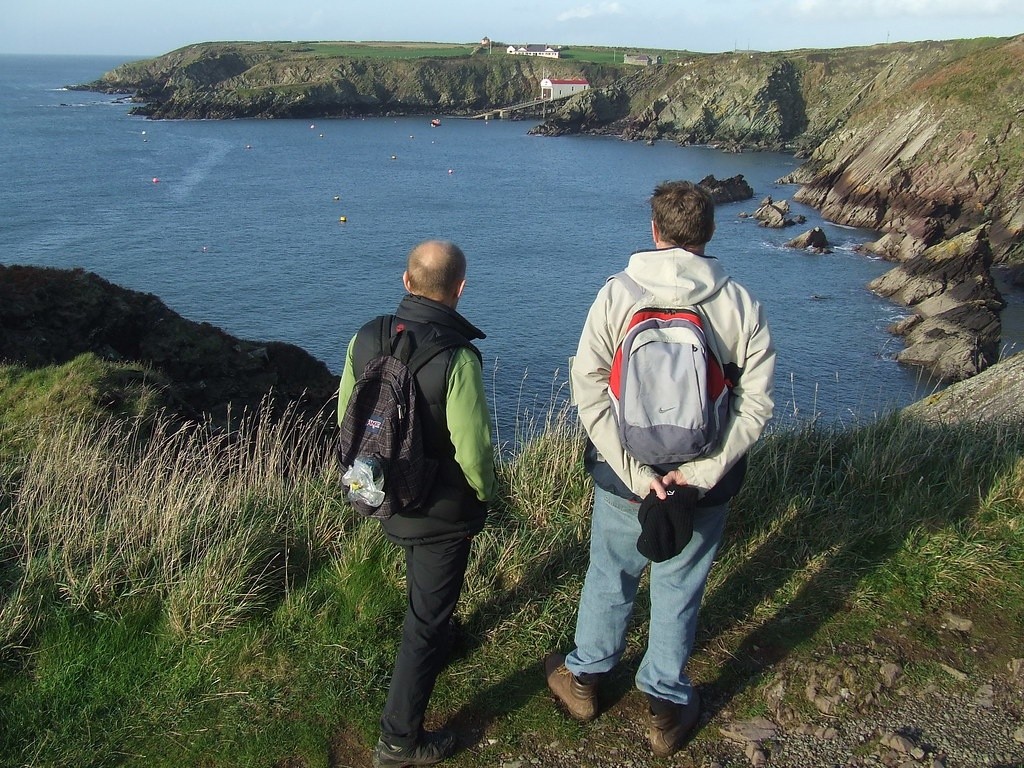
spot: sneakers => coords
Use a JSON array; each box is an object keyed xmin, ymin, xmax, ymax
[
  {"xmin": 543, "ymin": 654, "xmax": 598, "ymax": 721},
  {"xmin": 372, "ymin": 731, "xmax": 457, "ymax": 768},
  {"xmin": 648, "ymin": 687, "xmax": 700, "ymax": 759}
]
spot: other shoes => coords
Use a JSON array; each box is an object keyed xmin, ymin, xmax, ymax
[{"xmin": 448, "ymin": 619, "xmax": 455, "ymax": 647}]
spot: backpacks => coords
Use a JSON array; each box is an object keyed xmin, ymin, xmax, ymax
[
  {"xmin": 605, "ymin": 270, "xmax": 743, "ymax": 466},
  {"xmin": 336, "ymin": 315, "xmax": 483, "ymax": 520}
]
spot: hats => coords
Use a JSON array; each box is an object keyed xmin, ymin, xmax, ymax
[{"xmin": 636, "ymin": 485, "xmax": 699, "ymax": 563}]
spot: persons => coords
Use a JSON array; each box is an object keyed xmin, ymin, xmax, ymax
[
  {"xmin": 338, "ymin": 241, "xmax": 499, "ymax": 768},
  {"xmin": 545, "ymin": 181, "xmax": 775, "ymax": 757}
]
